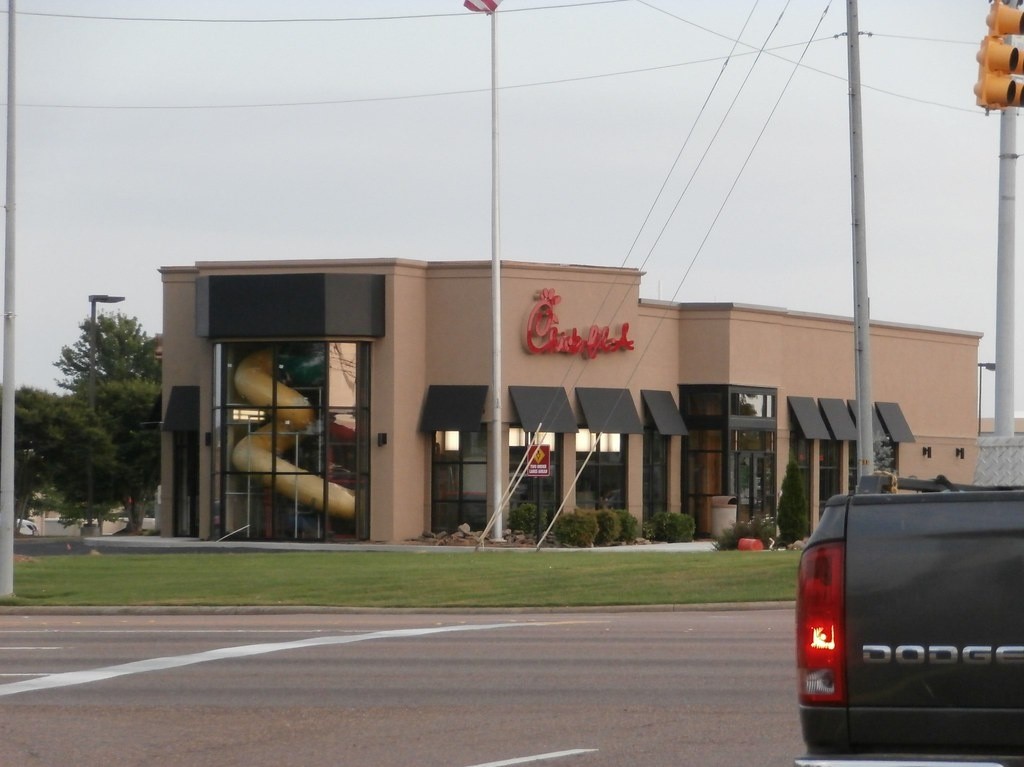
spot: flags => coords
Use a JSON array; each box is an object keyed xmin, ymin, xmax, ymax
[{"xmin": 464, "ymin": 0, "xmax": 504, "ymax": 15}]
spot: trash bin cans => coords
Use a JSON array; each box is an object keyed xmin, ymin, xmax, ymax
[{"xmin": 710, "ymin": 496, "xmax": 738, "ymax": 540}]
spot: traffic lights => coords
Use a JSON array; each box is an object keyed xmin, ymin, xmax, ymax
[{"xmin": 974, "ymin": 0, "xmax": 1024, "ymax": 114}]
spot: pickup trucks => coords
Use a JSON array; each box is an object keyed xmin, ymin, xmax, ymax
[{"xmin": 790, "ymin": 472, "xmax": 1024, "ymax": 765}]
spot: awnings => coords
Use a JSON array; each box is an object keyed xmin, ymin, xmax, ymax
[
  {"xmin": 641, "ymin": 390, "xmax": 689, "ymax": 435},
  {"xmin": 575, "ymin": 387, "xmax": 645, "ymax": 434},
  {"xmin": 875, "ymin": 402, "xmax": 916, "ymax": 443},
  {"xmin": 421, "ymin": 384, "xmax": 489, "ymax": 431},
  {"xmin": 818, "ymin": 398, "xmax": 857, "ymax": 440},
  {"xmin": 787, "ymin": 396, "xmax": 831, "ymax": 440},
  {"xmin": 162, "ymin": 386, "xmax": 200, "ymax": 431},
  {"xmin": 847, "ymin": 400, "xmax": 888, "ymax": 441},
  {"xmin": 508, "ymin": 385, "xmax": 579, "ymax": 432}
]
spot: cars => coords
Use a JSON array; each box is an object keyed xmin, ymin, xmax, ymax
[{"xmin": 15, "ymin": 519, "xmax": 38, "ymax": 536}]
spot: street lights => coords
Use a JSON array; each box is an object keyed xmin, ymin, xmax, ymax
[{"xmin": 84, "ymin": 295, "xmax": 126, "ymax": 527}]
